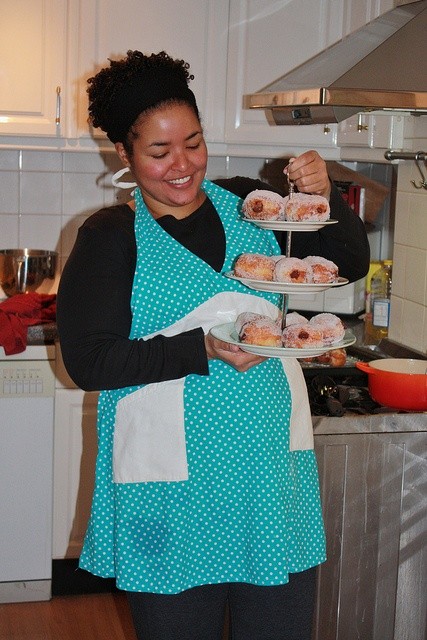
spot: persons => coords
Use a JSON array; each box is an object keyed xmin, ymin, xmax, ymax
[{"xmin": 57, "ymin": 51, "xmax": 370, "ymax": 638}]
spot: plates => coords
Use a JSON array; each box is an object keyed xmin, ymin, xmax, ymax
[
  {"xmin": 220, "ymin": 271, "xmax": 349, "ymax": 298},
  {"xmin": 241, "ymin": 216, "xmax": 340, "ymax": 236},
  {"xmin": 208, "ymin": 321, "xmax": 355, "ymax": 359}
]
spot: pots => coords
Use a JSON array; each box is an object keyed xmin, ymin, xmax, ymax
[
  {"xmin": 0, "ymin": 249, "xmax": 60, "ymax": 297},
  {"xmin": 352, "ymin": 358, "xmax": 426, "ymax": 411}
]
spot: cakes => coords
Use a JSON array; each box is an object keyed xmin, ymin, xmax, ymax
[
  {"xmin": 242, "ymin": 189, "xmax": 330, "ymax": 222},
  {"xmin": 235, "ymin": 311, "xmax": 346, "ymax": 349},
  {"xmin": 235, "ymin": 253, "xmax": 339, "ymax": 284}
]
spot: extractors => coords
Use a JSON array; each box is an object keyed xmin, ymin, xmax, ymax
[{"xmin": 244, "ymin": 1, "xmax": 427, "ymax": 128}]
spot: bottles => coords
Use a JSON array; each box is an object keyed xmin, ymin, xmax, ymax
[{"xmin": 365, "ymin": 254, "xmax": 392, "ymax": 333}]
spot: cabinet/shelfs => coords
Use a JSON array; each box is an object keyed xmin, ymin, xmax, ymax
[
  {"xmin": 53, "ymin": 392, "xmax": 101, "ymax": 560},
  {"xmin": 229, "ymin": 1, "xmax": 405, "ymax": 164},
  {"xmin": 385, "ymin": 149, "xmax": 426, "ymax": 189},
  {"xmin": 1, "ymin": 0, "xmax": 230, "ymax": 158}
]
